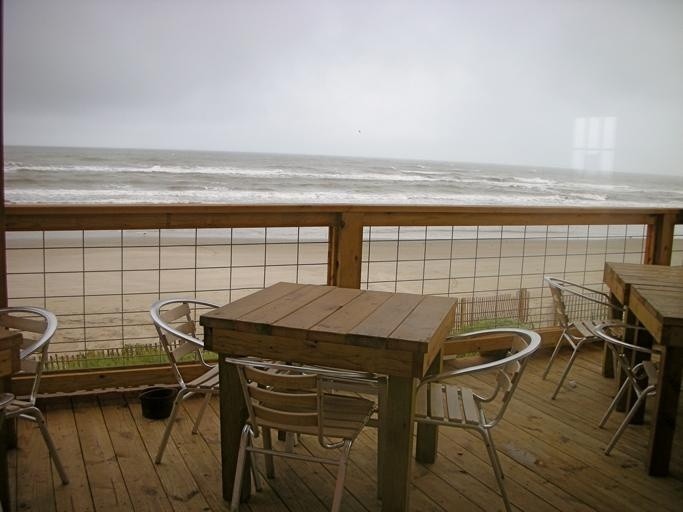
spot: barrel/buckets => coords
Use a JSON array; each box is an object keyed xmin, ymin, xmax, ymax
[{"xmin": 138, "ymin": 385, "xmax": 178, "ymax": 420}]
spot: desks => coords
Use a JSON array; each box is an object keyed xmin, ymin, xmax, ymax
[
  {"xmin": 198, "ymin": 281, "xmax": 458, "ymax": 512},
  {"xmin": 601, "ymin": 261, "xmax": 682, "ymax": 477}
]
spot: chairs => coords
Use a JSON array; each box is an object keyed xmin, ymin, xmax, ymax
[
  {"xmin": 542, "ymin": 277, "xmax": 682, "ymax": 456},
  {"xmin": 0, "ymin": 305, "xmax": 69, "ymax": 486}
]
